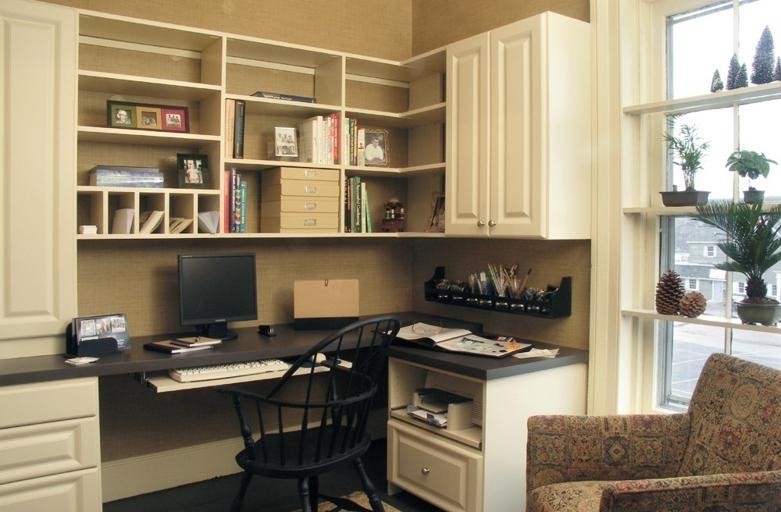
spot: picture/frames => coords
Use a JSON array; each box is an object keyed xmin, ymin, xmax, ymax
[{"xmin": 1, "ymin": 311, "xmax": 591, "ymax": 512}]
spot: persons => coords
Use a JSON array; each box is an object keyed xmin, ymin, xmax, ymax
[
  {"xmin": 142, "ymin": 112, "xmax": 156, "ymax": 126},
  {"xmin": 364, "ymin": 137, "xmax": 385, "ymax": 161},
  {"xmin": 185, "ymin": 163, "xmax": 202, "ymax": 183},
  {"xmin": 277, "ymin": 132, "xmax": 298, "ymax": 157},
  {"xmin": 167, "ymin": 115, "xmax": 181, "ymax": 127},
  {"xmin": 116, "ymin": 110, "xmax": 131, "ymax": 124},
  {"xmin": 113, "ymin": 319, "xmax": 125, "ymax": 329},
  {"xmin": 98, "ymin": 319, "xmax": 108, "ymax": 334}
]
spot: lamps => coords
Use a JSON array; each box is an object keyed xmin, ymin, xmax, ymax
[
  {"xmin": 212, "ymin": 315, "xmax": 402, "ymax": 511},
  {"xmin": 525, "ymin": 353, "xmax": 781, "ymax": 511}
]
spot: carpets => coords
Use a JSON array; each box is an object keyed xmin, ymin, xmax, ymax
[{"xmin": 298, "ymin": 488, "xmax": 403, "ymax": 512}]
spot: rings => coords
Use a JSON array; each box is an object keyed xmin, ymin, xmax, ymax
[{"xmin": 486, "ymin": 263, "xmax": 532, "ymax": 298}]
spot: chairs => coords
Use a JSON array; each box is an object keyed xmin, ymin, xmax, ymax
[
  {"xmin": 525, "ymin": 353, "xmax": 781, "ymax": 511},
  {"xmin": 212, "ymin": 315, "xmax": 402, "ymax": 511}
]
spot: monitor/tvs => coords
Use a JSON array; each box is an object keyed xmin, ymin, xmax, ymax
[
  {"xmin": 198, "ymin": 211, "xmax": 220, "ymax": 233},
  {"xmin": 344, "ymin": 175, "xmax": 372, "ymax": 234},
  {"xmin": 233, "ymin": 100, "xmax": 246, "ymax": 160},
  {"xmin": 298, "ymin": 112, "xmax": 365, "ymax": 165},
  {"xmin": 142, "ymin": 339, "xmax": 213, "ymax": 355},
  {"xmin": 224, "ymin": 98, "xmax": 235, "ymax": 159},
  {"xmin": 251, "ymin": 91, "xmax": 316, "ymax": 103},
  {"xmin": 170, "ymin": 335, "xmax": 224, "ymax": 348},
  {"xmin": 408, "ymin": 408, "xmax": 447, "ymax": 427},
  {"xmin": 139, "ymin": 210, "xmax": 165, "ymax": 234},
  {"xmin": 382, "ymin": 321, "xmax": 533, "ymax": 360},
  {"xmin": 89, "ymin": 165, "xmax": 165, "ymax": 187},
  {"xmin": 111, "ymin": 208, "xmax": 134, "ymax": 235},
  {"xmin": 225, "ymin": 167, "xmax": 248, "ymax": 233}
]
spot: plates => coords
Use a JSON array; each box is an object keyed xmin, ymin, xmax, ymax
[{"xmin": 618, "ymin": 0, "xmax": 781, "ymax": 335}]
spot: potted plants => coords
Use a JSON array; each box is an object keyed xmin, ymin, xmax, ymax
[
  {"xmin": 689, "ymin": 200, "xmax": 781, "ymax": 327},
  {"xmin": 661, "ymin": 110, "xmax": 713, "ymax": 208},
  {"xmin": 726, "ymin": 148, "xmax": 780, "ymax": 205}
]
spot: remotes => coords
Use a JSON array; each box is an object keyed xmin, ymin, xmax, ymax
[{"xmin": 310, "ymin": 352, "xmax": 325, "ymax": 365}]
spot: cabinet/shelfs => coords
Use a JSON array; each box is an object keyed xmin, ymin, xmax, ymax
[
  {"xmin": 3, "ymin": 2, "xmax": 78, "ymax": 360},
  {"xmin": 316, "ymin": 53, "xmax": 449, "ymax": 235},
  {"xmin": 618, "ymin": 0, "xmax": 781, "ymax": 335},
  {"xmin": 77, "ymin": 12, "xmax": 222, "ymax": 237},
  {"xmin": 446, "ymin": 12, "xmax": 593, "ymax": 240},
  {"xmin": 201, "ymin": 34, "xmax": 344, "ymax": 237}
]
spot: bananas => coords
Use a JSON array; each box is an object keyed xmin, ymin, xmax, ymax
[{"xmin": 168, "ymin": 359, "xmax": 290, "ymax": 383}]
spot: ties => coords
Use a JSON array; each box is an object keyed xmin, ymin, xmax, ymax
[{"xmin": 177, "ymin": 253, "xmax": 258, "ymax": 341}]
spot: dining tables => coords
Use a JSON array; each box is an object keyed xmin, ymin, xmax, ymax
[
  {"xmin": 726, "ymin": 148, "xmax": 780, "ymax": 205},
  {"xmin": 661, "ymin": 110, "xmax": 713, "ymax": 208},
  {"xmin": 689, "ymin": 200, "xmax": 781, "ymax": 327}
]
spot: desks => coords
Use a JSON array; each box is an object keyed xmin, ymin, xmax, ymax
[{"xmin": 1, "ymin": 311, "xmax": 591, "ymax": 512}]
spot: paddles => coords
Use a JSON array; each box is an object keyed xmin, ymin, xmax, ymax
[{"xmin": 298, "ymin": 488, "xmax": 403, "ymax": 512}]
